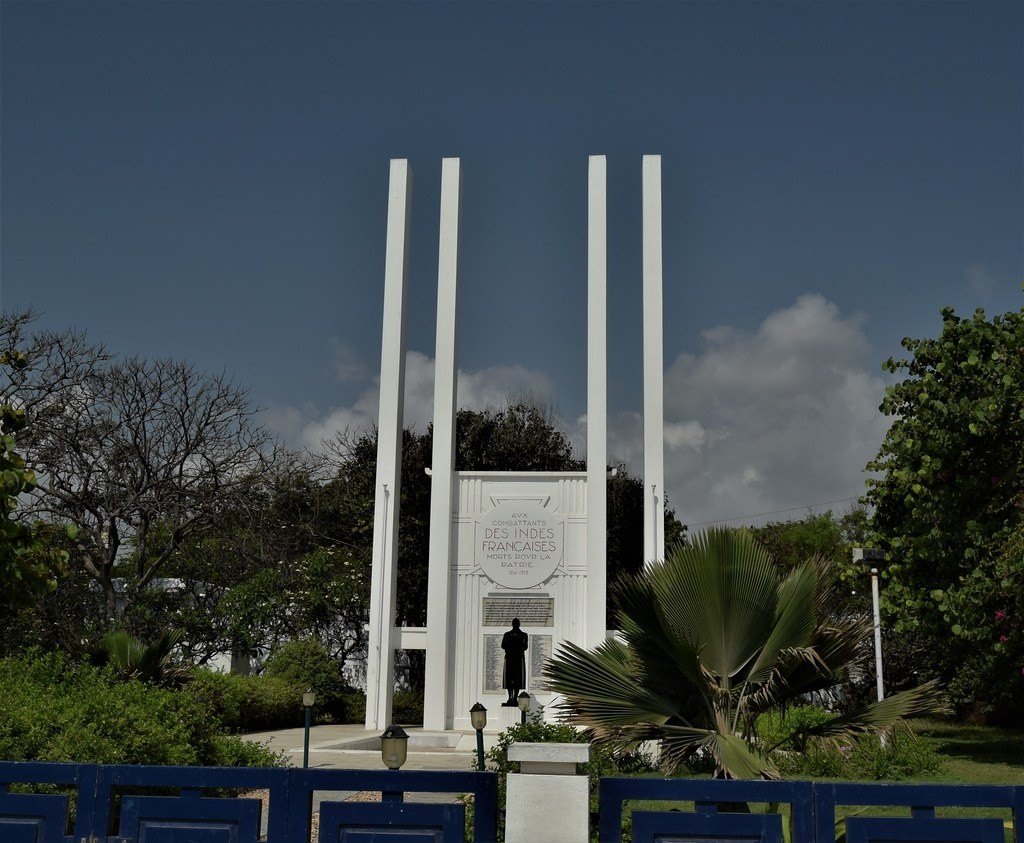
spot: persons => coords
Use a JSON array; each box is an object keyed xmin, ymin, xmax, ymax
[{"xmin": 501, "ymin": 617, "xmax": 528, "ymax": 707}]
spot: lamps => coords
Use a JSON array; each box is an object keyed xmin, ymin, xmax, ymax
[
  {"xmin": 469, "ymin": 702, "xmax": 488, "ymax": 731},
  {"xmin": 517, "ymin": 691, "xmax": 531, "ymax": 711},
  {"xmin": 302, "ymin": 687, "xmax": 316, "ymax": 706},
  {"xmin": 380, "ymin": 722, "xmax": 410, "ymax": 768}
]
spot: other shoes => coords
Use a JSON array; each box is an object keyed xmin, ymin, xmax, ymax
[{"xmin": 507, "ymin": 698, "xmax": 518, "ymax": 705}]
